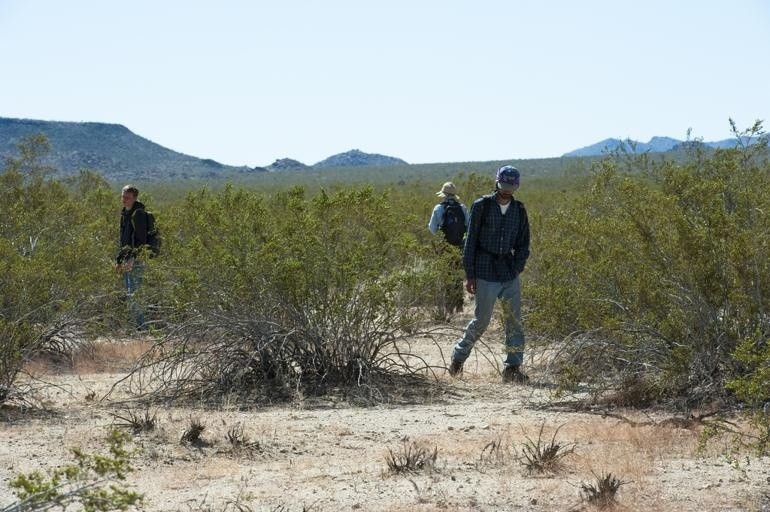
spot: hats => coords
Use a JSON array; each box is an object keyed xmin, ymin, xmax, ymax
[
  {"xmin": 436, "ymin": 181, "xmax": 460, "ymax": 200},
  {"xmin": 495, "ymin": 165, "xmax": 520, "ymax": 189}
]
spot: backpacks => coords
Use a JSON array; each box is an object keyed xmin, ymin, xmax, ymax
[
  {"xmin": 439, "ymin": 201, "xmax": 467, "ymax": 246},
  {"xmin": 131, "ymin": 208, "xmax": 162, "ymax": 259}
]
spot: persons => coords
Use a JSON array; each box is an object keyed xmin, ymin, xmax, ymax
[
  {"xmin": 444, "ymin": 162, "xmax": 533, "ymax": 387},
  {"xmin": 111, "ymin": 183, "xmax": 151, "ymax": 333},
  {"xmin": 426, "ymin": 179, "xmax": 471, "ymax": 318}
]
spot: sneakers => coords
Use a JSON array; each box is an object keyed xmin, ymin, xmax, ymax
[
  {"xmin": 501, "ymin": 368, "xmax": 531, "ymax": 383},
  {"xmin": 448, "ymin": 354, "xmax": 464, "ymax": 380}
]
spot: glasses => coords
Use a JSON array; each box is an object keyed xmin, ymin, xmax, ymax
[{"xmin": 497, "ymin": 183, "xmax": 517, "ymax": 191}]
